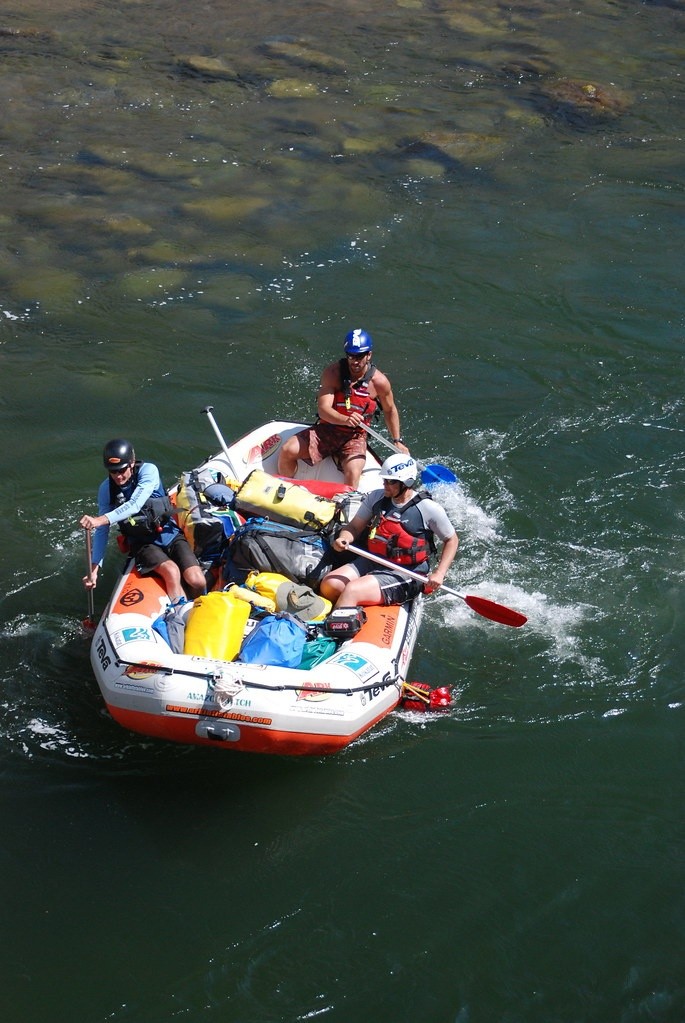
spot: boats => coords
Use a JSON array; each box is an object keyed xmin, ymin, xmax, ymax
[{"xmin": 87, "ymin": 419, "xmax": 427, "ymax": 756}]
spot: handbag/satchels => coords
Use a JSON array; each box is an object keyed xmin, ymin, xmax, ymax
[{"xmin": 155, "ymin": 466, "xmax": 368, "ymax": 670}]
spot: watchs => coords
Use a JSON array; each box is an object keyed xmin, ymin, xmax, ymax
[{"xmin": 393, "ymin": 438, "xmax": 403, "ymax": 443}]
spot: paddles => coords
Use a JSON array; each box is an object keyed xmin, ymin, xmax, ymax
[
  {"xmin": 201, "ymin": 405, "xmax": 246, "ymax": 484},
  {"xmin": 357, "ymin": 421, "xmax": 458, "ymax": 485},
  {"xmin": 81, "ymin": 528, "xmax": 100, "ymax": 629},
  {"xmin": 344, "ymin": 542, "xmax": 528, "ymax": 628}
]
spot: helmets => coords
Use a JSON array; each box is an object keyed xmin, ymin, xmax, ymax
[
  {"xmin": 380, "ymin": 453, "xmax": 416, "ymax": 488},
  {"xmin": 103, "ymin": 439, "xmax": 134, "ymax": 470},
  {"xmin": 344, "ymin": 329, "xmax": 372, "ymax": 354}
]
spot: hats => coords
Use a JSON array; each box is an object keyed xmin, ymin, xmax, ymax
[{"xmin": 277, "ymin": 582, "xmax": 326, "ymax": 621}]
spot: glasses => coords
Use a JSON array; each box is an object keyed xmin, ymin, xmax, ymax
[
  {"xmin": 384, "ymin": 479, "xmax": 398, "ymax": 484},
  {"xmin": 109, "ymin": 465, "xmax": 129, "ymax": 474},
  {"xmin": 346, "ymin": 354, "xmax": 366, "ymax": 360}
]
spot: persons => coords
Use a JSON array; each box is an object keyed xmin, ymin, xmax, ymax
[
  {"xmin": 319, "ymin": 452, "xmax": 460, "ymax": 612},
  {"xmin": 79, "ymin": 438, "xmax": 208, "ymax": 606},
  {"xmin": 277, "ymin": 328, "xmax": 411, "ymax": 491}
]
